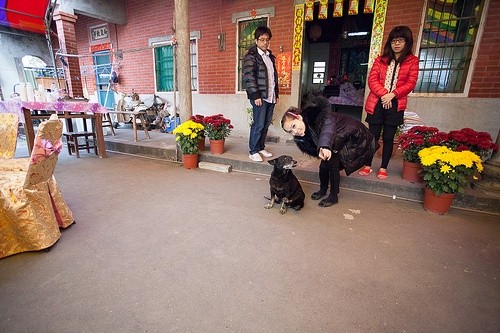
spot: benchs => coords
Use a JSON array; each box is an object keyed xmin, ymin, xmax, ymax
[{"xmin": 101, "ymin": 110, "xmax": 150, "ymax": 143}]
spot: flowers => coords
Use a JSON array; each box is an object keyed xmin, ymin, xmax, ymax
[
  {"xmin": 416, "ymin": 146, "xmax": 485, "ymax": 197},
  {"xmin": 189, "ymin": 114, "xmax": 205, "ymax": 137},
  {"xmin": 398, "ymin": 127, "xmax": 448, "ymax": 163},
  {"xmin": 203, "ymin": 114, "xmax": 234, "ymax": 140},
  {"xmin": 446, "ymin": 127, "xmax": 496, "ymax": 180},
  {"xmin": 172, "ymin": 120, "xmax": 205, "ymax": 154}
]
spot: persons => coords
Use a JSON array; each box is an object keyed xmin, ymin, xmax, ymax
[
  {"xmin": 281, "ymin": 96, "xmax": 376, "ymax": 207},
  {"xmin": 359, "ymin": 26, "xmax": 419, "ymax": 179},
  {"xmin": 242, "ymin": 27, "xmax": 279, "ymax": 162}
]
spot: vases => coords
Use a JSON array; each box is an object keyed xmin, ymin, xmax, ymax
[
  {"xmin": 183, "ymin": 153, "xmax": 199, "ymax": 168},
  {"xmin": 209, "ymin": 138, "xmax": 225, "ymax": 154},
  {"xmin": 198, "ymin": 137, "xmax": 206, "ymax": 151},
  {"xmin": 402, "ymin": 161, "xmax": 426, "ymax": 184},
  {"xmin": 423, "ymin": 186, "xmax": 456, "ymax": 215}
]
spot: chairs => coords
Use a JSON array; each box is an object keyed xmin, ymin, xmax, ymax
[
  {"xmin": 0, "ymin": 112, "xmax": 98, "ymax": 258},
  {"xmin": 328, "ymin": 81, "xmax": 366, "ymax": 119}
]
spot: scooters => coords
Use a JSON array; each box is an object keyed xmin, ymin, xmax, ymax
[{"xmin": 116, "ymin": 89, "xmax": 168, "ymax": 131}]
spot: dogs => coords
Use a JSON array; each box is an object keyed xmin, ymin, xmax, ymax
[{"xmin": 264, "ymin": 155, "xmax": 305, "ymax": 214}]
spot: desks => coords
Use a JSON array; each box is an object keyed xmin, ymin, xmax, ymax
[{"xmin": 0, "ymin": 100, "xmax": 107, "ymax": 160}]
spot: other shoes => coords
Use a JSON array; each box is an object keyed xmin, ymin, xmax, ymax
[
  {"xmin": 259, "ymin": 150, "xmax": 273, "ymax": 157},
  {"xmin": 318, "ymin": 198, "xmax": 338, "ymax": 207},
  {"xmin": 311, "ymin": 191, "xmax": 326, "ymax": 200},
  {"xmin": 249, "ymin": 153, "xmax": 263, "ymax": 161}
]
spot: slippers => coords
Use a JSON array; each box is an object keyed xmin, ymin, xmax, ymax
[
  {"xmin": 377, "ymin": 171, "xmax": 388, "ymax": 179},
  {"xmin": 358, "ymin": 168, "xmax": 373, "ymax": 176}
]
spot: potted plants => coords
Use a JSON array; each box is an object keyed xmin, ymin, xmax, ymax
[{"xmin": 378, "ymin": 125, "xmax": 405, "ymax": 158}]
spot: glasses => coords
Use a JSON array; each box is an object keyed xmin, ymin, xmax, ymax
[
  {"xmin": 257, "ymin": 38, "xmax": 270, "ymax": 42},
  {"xmin": 390, "ymin": 38, "xmax": 405, "ymax": 44}
]
